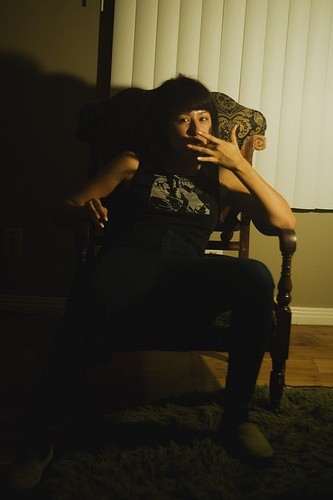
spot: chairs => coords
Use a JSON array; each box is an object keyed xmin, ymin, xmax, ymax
[{"xmin": 51, "ymin": 84, "xmax": 299, "ymax": 415}]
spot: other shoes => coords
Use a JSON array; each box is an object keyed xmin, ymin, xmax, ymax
[
  {"xmin": 219, "ymin": 414, "xmax": 273, "ymax": 460},
  {"xmin": 6, "ymin": 443, "xmax": 53, "ymax": 490}
]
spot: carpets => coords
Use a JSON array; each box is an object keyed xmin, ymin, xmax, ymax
[{"xmin": 0, "ymin": 383, "xmax": 333, "ymax": 500}]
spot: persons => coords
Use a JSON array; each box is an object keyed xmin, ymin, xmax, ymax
[{"xmin": 5, "ymin": 74, "xmax": 294, "ymax": 491}]
